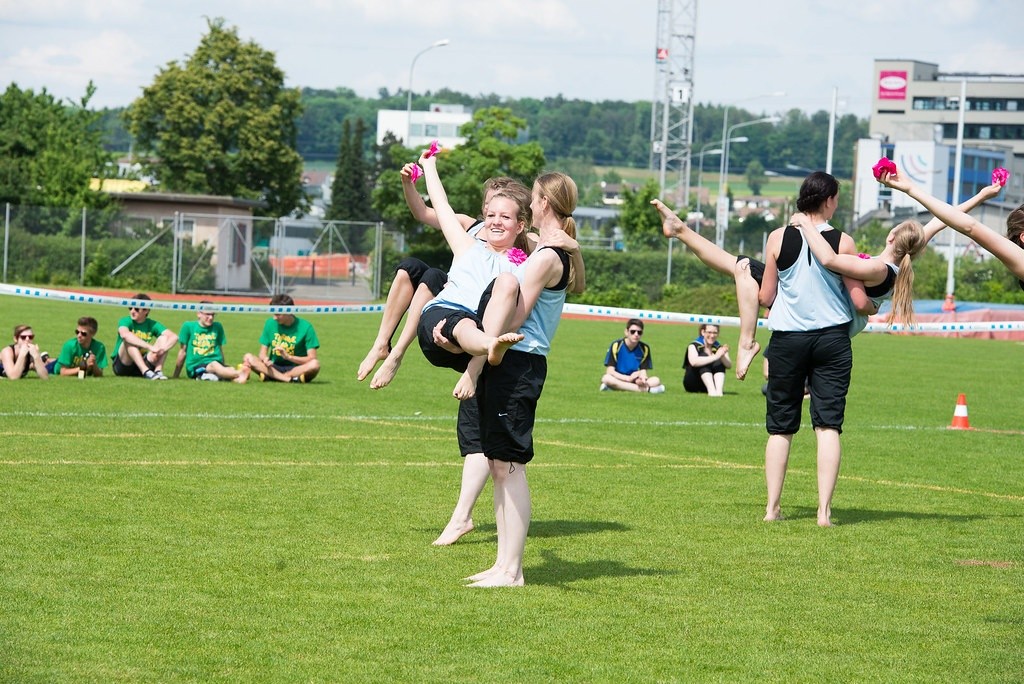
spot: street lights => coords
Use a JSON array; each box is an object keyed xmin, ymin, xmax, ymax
[
  {"xmin": 716, "ymin": 115, "xmax": 782, "ymax": 252},
  {"xmin": 404, "ymin": 39, "xmax": 452, "ymax": 146},
  {"xmin": 694, "ymin": 135, "xmax": 749, "ymax": 234}
]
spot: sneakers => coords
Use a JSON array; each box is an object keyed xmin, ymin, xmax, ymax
[
  {"xmin": 145, "ymin": 370, "xmax": 158, "ymax": 379},
  {"xmin": 158, "ymin": 372, "xmax": 167, "ymax": 379}
]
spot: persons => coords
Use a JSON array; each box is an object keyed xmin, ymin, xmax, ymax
[
  {"xmin": 682, "ymin": 324, "xmax": 732, "ymax": 397},
  {"xmin": 243, "ymin": 294, "xmax": 320, "ymax": 382},
  {"xmin": 650, "ymin": 166, "xmax": 1010, "ymax": 381},
  {"xmin": 758, "ymin": 171, "xmax": 878, "ymax": 527},
  {"xmin": 0, "ymin": 325, "xmax": 50, "ymax": 380},
  {"xmin": 169, "ymin": 300, "xmax": 252, "ymax": 384},
  {"xmin": 111, "ymin": 294, "xmax": 179, "ymax": 380},
  {"xmin": 356, "ymin": 142, "xmax": 587, "ymax": 588},
  {"xmin": 599, "ymin": 318, "xmax": 666, "ymax": 394},
  {"xmin": 30, "ymin": 317, "xmax": 110, "ymax": 379},
  {"xmin": 872, "ymin": 157, "xmax": 1024, "ymax": 294}
]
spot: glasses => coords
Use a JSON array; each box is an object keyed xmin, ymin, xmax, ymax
[
  {"xmin": 129, "ymin": 307, "xmax": 148, "ymax": 312},
  {"xmin": 75, "ymin": 329, "xmax": 88, "ymax": 337},
  {"xmin": 629, "ymin": 330, "xmax": 643, "ymax": 336},
  {"xmin": 19, "ymin": 335, "xmax": 34, "ymax": 340}
]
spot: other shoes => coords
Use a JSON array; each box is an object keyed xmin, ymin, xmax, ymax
[
  {"xmin": 294, "ymin": 374, "xmax": 307, "ymax": 384},
  {"xmin": 41, "ymin": 352, "xmax": 49, "ymax": 361},
  {"xmin": 259, "ymin": 372, "xmax": 270, "ymax": 381}
]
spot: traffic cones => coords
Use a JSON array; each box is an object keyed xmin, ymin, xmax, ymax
[{"xmin": 946, "ymin": 393, "xmax": 976, "ymax": 430}]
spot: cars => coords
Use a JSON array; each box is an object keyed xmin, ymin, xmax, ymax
[{"xmin": 320, "ymin": 172, "xmax": 334, "ymax": 207}]
